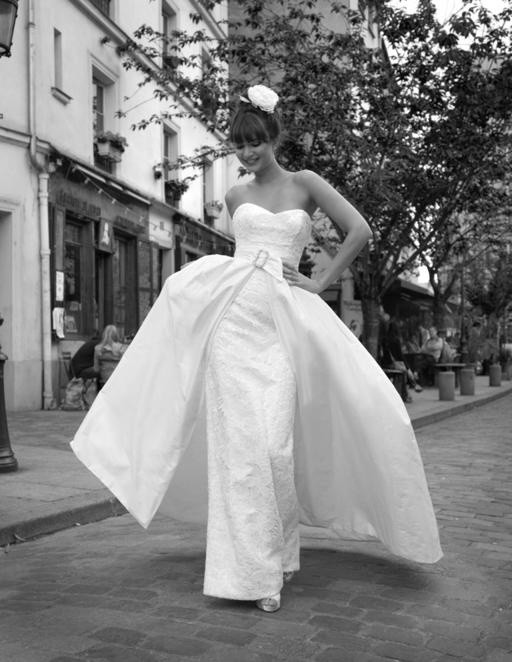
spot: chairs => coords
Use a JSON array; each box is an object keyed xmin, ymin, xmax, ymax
[{"xmin": 61, "ymin": 351, "xmax": 120, "ymax": 409}]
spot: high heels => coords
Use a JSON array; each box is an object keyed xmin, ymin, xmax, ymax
[{"xmin": 255, "ymin": 572, "xmax": 295, "ymax": 613}]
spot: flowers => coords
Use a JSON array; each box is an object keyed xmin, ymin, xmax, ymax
[{"xmin": 248, "ymin": 83, "xmax": 279, "ymax": 113}]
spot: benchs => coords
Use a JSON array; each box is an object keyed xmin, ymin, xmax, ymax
[
  {"xmin": 432, "ymin": 362, "xmax": 466, "ymax": 370},
  {"xmin": 382, "ymin": 368, "xmax": 407, "ymax": 402}
]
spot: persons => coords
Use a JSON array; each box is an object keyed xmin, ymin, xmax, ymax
[
  {"xmin": 347, "ymin": 319, "xmax": 361, "ymax": 339},
  {"xmin": 92, "ymin": 324, "xmax": 128, "ymax": 388},
  {"xmin": 65, "ymin": 77, "xmax": 446, "ymax": 617},
  {"xmin": 381, "ymin": 312, "xmax": 483, "ymax": 403},
  {"xmin": 68, "ymin": 329, "xmax": 102, "ymax": 394}
]
